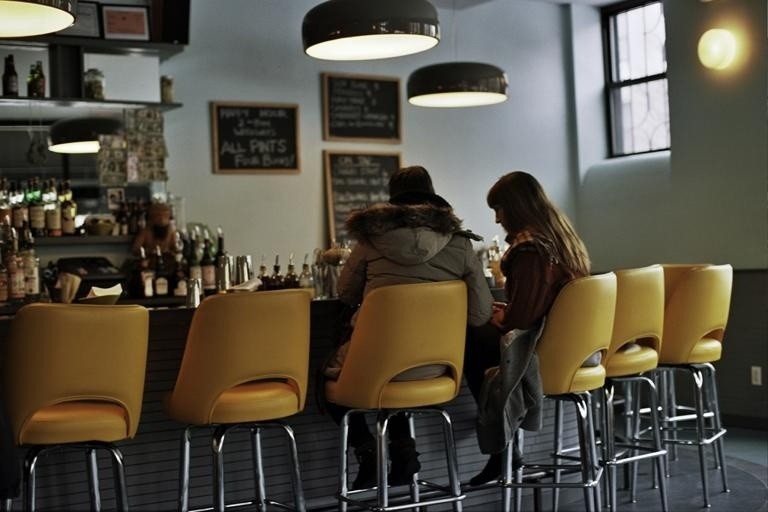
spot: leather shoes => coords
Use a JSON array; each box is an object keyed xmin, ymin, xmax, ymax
[{"xmin": 468, "ymin": 446, "xmax": 527, "ymax": 487}]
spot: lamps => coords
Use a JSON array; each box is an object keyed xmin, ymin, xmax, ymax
[
  {"xmin": 407, "ymin": 63, "xmax": 509, "ymax": 109},
  {"xmin": 698, "ymin": 29, "xmax": 737, "ymax": 70},
  {"xmin": 303, "ymin": 0, "xmax": 440, "ymax": 62},
  {"xmin": 48, "ymin": 114, "xmax": 125, "ymax": 155},
  {"xmin": 0, "ymin": 0, "xmax": 77, "ymax": 39}
]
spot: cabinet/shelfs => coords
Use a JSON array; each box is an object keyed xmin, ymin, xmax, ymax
[{"xmin": 1, "ymin": 0, "xmax": 192, "ymax": 132}]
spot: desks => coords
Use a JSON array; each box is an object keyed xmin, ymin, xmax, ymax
[{"xmin": 2, "ymin": 268, "xmax": 612, "ymax": 508}]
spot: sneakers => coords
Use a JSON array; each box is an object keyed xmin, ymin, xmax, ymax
[
  {"xmin": 350, "ymin": 447, "xmax": 393, "ymax": 490},
  {"xmin": 388, "ymin": 441, "xmax": 421, "ymax": 486}
]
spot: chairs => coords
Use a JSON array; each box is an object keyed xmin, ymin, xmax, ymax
[
  {"xmin": 504, "ymin": 273, "xmax": 618, "ymax": 511},
  {"xmin": 553, "ymin": 262, "xmax": 667, "ymax": 511},
  {"xmin": 329, "ymin": 281, "xmax": 466, "ymax": 512},
  {"xmin": 165, "ymin": 289, "xmax": 312, "ymax": 512},
  {"xmin": 619, "ymin": 264, "xmax": 734, "ymax": 508},
  {"xmin": 2, "ymin": 304, "xmax": 150, "ymax": 511}
]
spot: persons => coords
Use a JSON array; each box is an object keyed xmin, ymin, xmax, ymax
[
  {"xmin": 313, "ymin": 165, "xmax": 495, "ymax": 490},
  {"xmin": 131, "ymin": 203, "xmax": 179, "ymax": 255},
  {"xmin": 464, "ymin": 171, "xmax": 593, "ymax": 485}
]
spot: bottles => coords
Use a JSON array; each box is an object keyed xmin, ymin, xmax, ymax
[
  {"xmin": 0, "ymin": 177, "xmax": 12, "ymax": 229},
  {"xmin": 172, "ymin": 232, "xmax": 189, "ymax": 296},
  {"xmin": 187, "ymin": 230, "xmax": 203, "ymax": 299},
  {"xmin": 26, "ymin": 178, "xmax": 32, "ymax": 203},
  {"xmin": 269, "ymin": 253, "xmax": 285, "ymax": 290},
  {"xmin": 2, "ymin": 54, "xmax": 19, "ymax": 97},
  {"xmin": 155, "ymin": 245, "xmax": 170, "ymax": 296},
  {"xmin": 256, "ymin": 254, "xmax": 270, "ymax": 291},
  {"xmin": 9, "ymin": 226, "xmax": 25, "ymax": 299},
  {"xmin": 45, "ymin": 176, "xmax": 62, "ymax": 237},
  {"xmin": 139, "ymin": 245, "xmax": 155, "ymax": 298},
  {"xmin": 57, "ymin": 180, "xmax": 65, "ymax": 203},
  {"xmin": 201, "ymin": 229, "xmax": 217, "ymax": 298},
  {"xmin": 299, "ymin": 252, "xmax": 315, "ymax": 297},
  {"xmin": 284, "ymin": 253, "xmax": 299, "ymax": 289},
  {"xmin": 42, "ymin": 180, "xmax": 49, "ymax": 204},
  {"xmin": 84, "ymin": 67, "xmax": 105, "ymax": 99},
  {"xmin": 160, "ymin": 74, "xmax": 175, "ymax": 103},
  {"xmin": 215, "ymin": 226, "xmax": 226, "ymax": 293},
  {"xmin": 28, "ymin": 176, "xmax": 47, "ymax": 238},
  {"xmin": 33, "ymin": 60, "xmax": 46, "ymax": 98},
  {"xmin": 12, "ymin": 181, "xmax": 31, "ymax": 236},
  {"xmin": 136, "ymin": 196, "xmax": 147, "ymax": 233},
  {"xmin": 115, "ymin": 202, "xmax": 129, "ymax": 235},
  {"xmin": 26, "ymin": 63, "xmax": 36, "ymax": 97},
  {"xmin": 61, "ymin": 181, "xmax": 79, "ymax": 236},
  {"xmin": 129, "ymin": 202, "xmax": 139, "ymax": 233},
  {"xmin": 20, "ymin": 227, "xmax": 41, "ymax": 298},
  {"xmin": 0, "ymin": 240, "xmax": 9, "ymax": 303}
]
